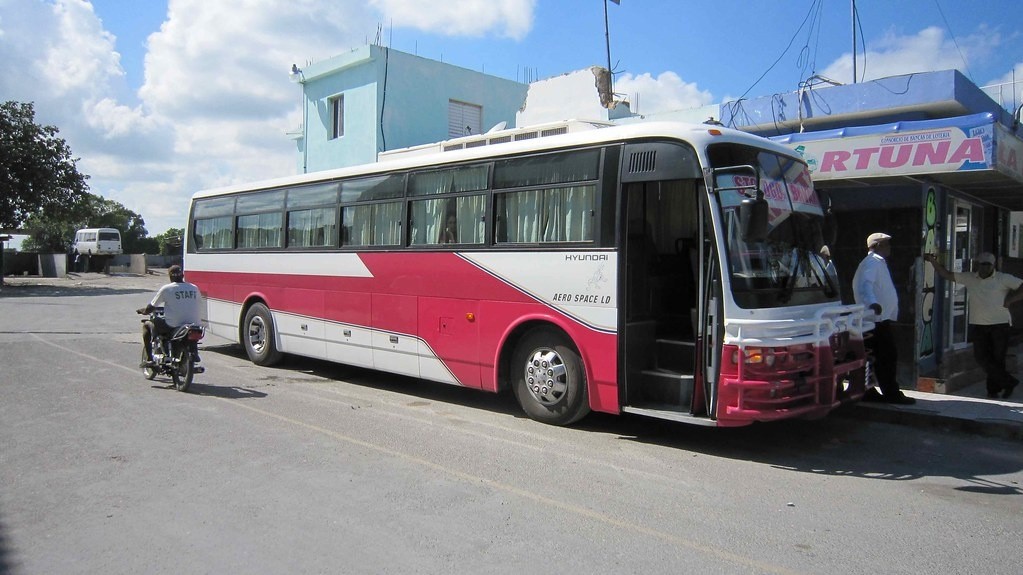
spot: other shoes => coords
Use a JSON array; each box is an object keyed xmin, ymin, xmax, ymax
[
  {"xmin": 861, "ymin": 387, "xmax": 884, "ymax": 402},
  {"xmin": 881, "ymin": 391, "xmax": 916, "ymax": 405},
  {"xmin": 139, "ymin": 360, "xmax": 156, "ymax": 368},
  {"xmin": 191, "ymin": 351, "xmax": 201, "ymax": 362},
  {"xmin": 988, "ymin": 392, "xmax": 998, "ymax": 398},
  {"xmin": 1002, "ymin": 378, "xmax": 1019, "ymax": 398}
]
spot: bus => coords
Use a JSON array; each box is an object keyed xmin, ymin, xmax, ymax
[{"xmin": 182, "ymin": 120, "xmax": 878, "ymax": 427}]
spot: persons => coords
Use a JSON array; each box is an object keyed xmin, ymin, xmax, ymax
[
  {"xmin": 139, "ymin": 265, "xmax": 203, "ymax": 367},
  {"xmin": 850, "ymin": 233, "xmax": 917, "ymax": 406},
  {"xmin": 927, "ymin": 251, "xmax": 1023, "ymax": 400}
]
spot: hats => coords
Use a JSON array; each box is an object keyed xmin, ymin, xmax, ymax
[
  {"xmin": 867, "ymin": 233, "xmax": 892, "ymax": 248},
  {"xmin": 976, "ymin": 252, "xmax": 995, "ymax": 265},
  {"xmin": 820, "ymin": 246, "xmax": 831, "ymax": 258},
  {"xmin": 166, "ymin": 265, "xmax": 183, "ymax": 276}
]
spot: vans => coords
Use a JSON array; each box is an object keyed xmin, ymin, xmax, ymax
[{"xmin": 73, "ymin": 228, "xmax": 124, "ymax": 261}]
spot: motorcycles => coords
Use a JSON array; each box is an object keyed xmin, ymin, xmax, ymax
[{"xmin": 136, "ymin": 306, "xmax": 206, "ymax": 393}]
